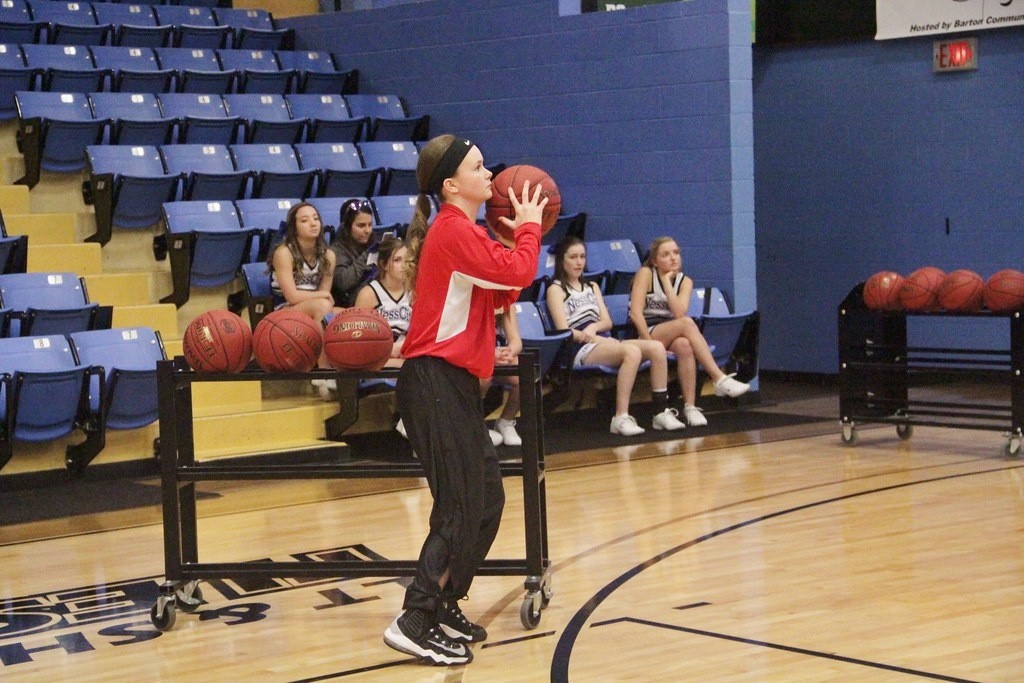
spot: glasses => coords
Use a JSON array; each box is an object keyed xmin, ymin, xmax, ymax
[{"xmin": 344, "ymin": 200, "xmax": 371, "ymax": 222}]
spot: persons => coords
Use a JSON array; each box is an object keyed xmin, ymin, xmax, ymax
[
  {"xmin": 479, "ymin": 305, "xmax": 523, "ymax": 446},
  {"xmin": 384, "ymin": 134, "xmax": 549, "ymax": 667},
  {"xmin": 355, "ymin": 238, "xmax": 418, "ymax": 458},
  {"xmin": 546, "ymin": 236, "xmax": 686, "ymax": 436},
  {"xmin": 268, "ymin": 202, "xmax": 346, "ymax": 402},
  {"xmin": 329, "ymin": 199, "xmax": 393, "ymax": 308},
  {"xmin": 626, "ymin": 236, "xmax": 750, "ymax": 427}
]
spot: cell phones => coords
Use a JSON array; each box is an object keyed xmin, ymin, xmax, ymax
[{"xmin": 382, "ymin": 232, "xmax": 393, "ymax": 242}]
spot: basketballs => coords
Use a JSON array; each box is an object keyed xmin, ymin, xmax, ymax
[
  {"xmin": 252, "ymin": 309, "xmax": 323, "ymax": 372},
  {"xmin": 938, "ymin": 270, "xmax": 985, "ymax": 310},
  {"xmin": 182, "ymin": 309, "xmax": 252, "ymax": 374},
  {"xmin": 486, "ymin": 164, "xmax": 562, "ymax": 242},
  {"xmin": 323, "ymin": 307, "xmax": 393, "ymax": 371},
  {"xmin": 914, "ymin": 267, "xmax": 941, "ymax": 271},
  {"xmin": 983, "ymin": 269, "xmax": 1024, "ymax": 310},
  {"xmin": 900, "ymin": 271, "xmax": 944, "ymax": 311},
  {"xmin": 863, "ymin": 271, "xmax": 904, "ymax": 310}
]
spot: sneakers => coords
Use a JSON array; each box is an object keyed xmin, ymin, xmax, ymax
[
  {"xmin": 652, "ymin": 407, "xmax": 685, "ymax": 430},
  {"xmin": 610, "ymin": 412, "xmax": 646, "ymax": 436},
  {"xmin": 436, "ymin": 595, "xmax": 487, "ymax": 643},
  {"xmin": 683, "ymin": 402, "xmax": 708, "ymax": 426},
  {"xmin": 712, "ymin": 373, "xmax": 751, "ymax": 397},
  {"xmin": 488, "ymin": 429, "xmax": 503, "ymax": 447},
  {"xmin": 383, "ymin": 607, "xmax": 475, "ymax": 666},
  {"xmin": 494, "ymin": 419, "xmax": 521, "ymax": 446}
]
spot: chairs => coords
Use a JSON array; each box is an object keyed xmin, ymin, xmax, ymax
[{"xmin": 0, "ymin": 0, "xmax": 759, "ymax": 470}]
